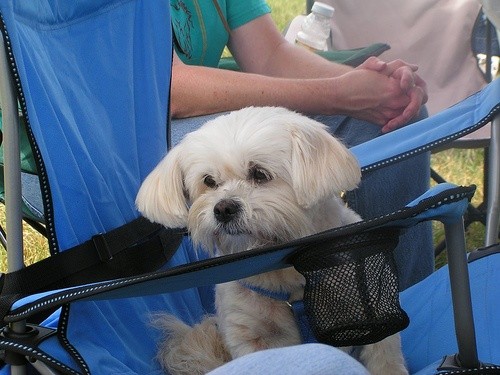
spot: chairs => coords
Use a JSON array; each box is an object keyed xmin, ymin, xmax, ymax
[
  {"xmin": 307, "ymin": 0, "xmax": 499, "ymax": 249},
  {"xmin": 0, "ymin": 0, "xmax": 500, "ymax": 375}
]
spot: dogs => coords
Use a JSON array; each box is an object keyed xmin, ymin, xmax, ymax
[{"xmin": 131, "ymin": 104, "xmax": 410, "ymax": 375}]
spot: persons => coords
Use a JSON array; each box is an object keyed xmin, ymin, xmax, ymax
[{"xmin": 0, "ymin": 3, "xmax": 439, "ymax": 293}]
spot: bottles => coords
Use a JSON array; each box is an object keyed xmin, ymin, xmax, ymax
[{"xmin": 294, "ymin": 1, "xmax": 337, "ymax": 53}]
